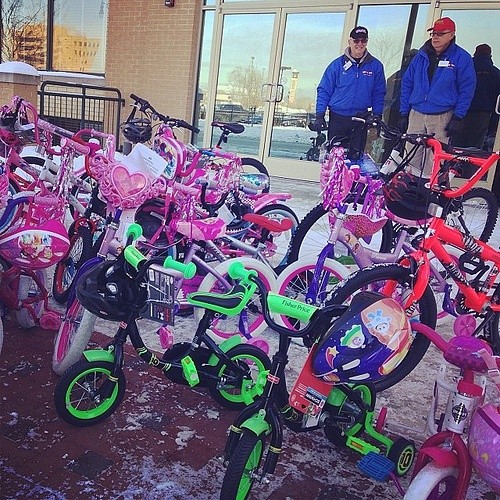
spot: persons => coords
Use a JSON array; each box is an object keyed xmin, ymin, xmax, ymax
[
  {"xmin": 450, "ymin": 44, "xmax": 500, "ymax": 177},
  {"xmin": 383, "ymin": 48, "xmax": 418, "ymax": 165},
  {"xmin": 314, "ymin": 25, "xmax": 386, "ymax": 160},
  {"xmin": 398, "ymin": 17, "xmax": 477, "ymax": 175}
]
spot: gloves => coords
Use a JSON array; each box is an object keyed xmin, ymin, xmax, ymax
[
  {"xmin": 372, "ymin": 115, "xmax": 382, "ymax": 136},
  {"xmin": 398, "ymin": 116, "xmax": 408, "ymax": 133},
  {"xmin": 314, "ymin": 115, "xmax": 327, "ymax": 135},
  {"xmin": 444, "ymin": 115, "xmax": 463, "ymax": 137}
]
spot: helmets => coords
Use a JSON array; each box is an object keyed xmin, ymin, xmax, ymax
[
  {"xmin": 0, "ymin": 219, "xmax": 70, "ymax": 271},
  {"xmin": 121, "ymin": 118, "xmax": 152, "ymax": 145},
  {"xmin": 0, "ymin": 117, "xmax": 26, "ymax": 158},
  {"xmin": 383, "ymin": 173, "xmax": 435, "ymax": 226},
  {"xmin": 328, "ymin": 211, "xmax": 388, "ymax": 238},
  {"xmin": 312, "ymin": 291, "xmax": 414, "ymax": 385},
  {"xmin": 468, "ymin": 404, "xmax": 500, "ymax": 497},
  {"xmin": 74, "ymin": 256, "xmax": 148, "ymax": 322}
]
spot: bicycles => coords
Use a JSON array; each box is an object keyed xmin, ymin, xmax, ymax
[
  {"xmin": 0, "ymin": 89, "xmax": 499, "ymax": 499},
  {"xmin": 299, "ymin": 132, "xmax": 326, "ymax": 162}
]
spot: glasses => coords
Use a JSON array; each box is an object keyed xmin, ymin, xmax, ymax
[
  {"xmin": 430, "ymin": 32, "xmax": 448, "ymax": 37},
  {"xmin": 353, "ymin": 40, "xmax": 368, "ymax": 43}
]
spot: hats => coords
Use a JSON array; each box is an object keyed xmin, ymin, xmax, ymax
[
  {"xmin": 350, "ymin": 26, "xmax": 368, "ymax": 39},
  {"xmin": 473, "ymin": 44, "xmax": 492, "ymax": 57},
  {"xmin": 405, "ymin": 48, "xmax": 418, "ymax": 56},
  {"xmin": 426, "ymin": 17, "xmax": 455, "ymax": 32}
]
